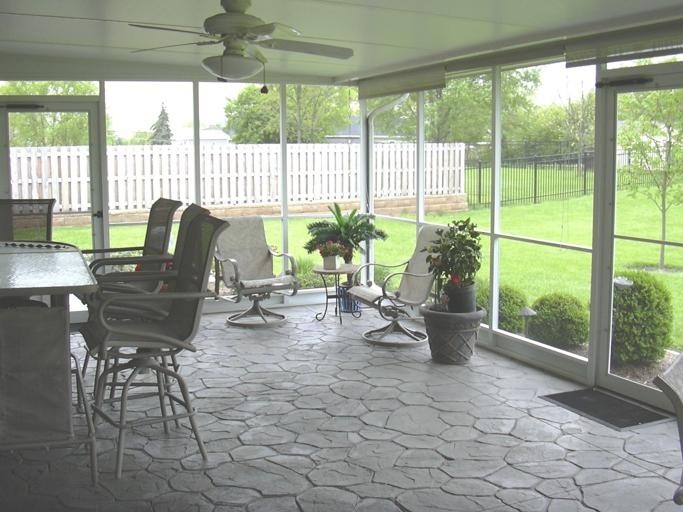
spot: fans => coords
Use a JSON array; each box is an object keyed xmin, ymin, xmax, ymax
[{"xmin": 126, "ymin": 0, "xmax": 354, "ymax": 95}]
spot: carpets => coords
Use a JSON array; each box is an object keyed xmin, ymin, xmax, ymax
[{"xmin": 536, "ymin": 387, "xmax": 677, "ymax": 434}]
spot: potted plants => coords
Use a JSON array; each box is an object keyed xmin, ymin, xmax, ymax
[
  {"xmin": 302, "ymin": 203, "xmax": 388, "ymax": 313},
  {"xmin": 417, "ymin": 217, "xmax": 487, "ymax": 365}
]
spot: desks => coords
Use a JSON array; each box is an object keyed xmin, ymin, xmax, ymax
[{"xmin": 311, "ymin": 263, "xmax": 362, "ymax": 324}]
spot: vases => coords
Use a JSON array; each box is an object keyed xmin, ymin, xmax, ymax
[{"xmin": 322, "ymin": 255, "xmax": 339, "ymax": 270}]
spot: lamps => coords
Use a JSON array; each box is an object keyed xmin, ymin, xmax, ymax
[{"xmin": 199, "ymin": 39, "xmax": 267, "ymax": 81}]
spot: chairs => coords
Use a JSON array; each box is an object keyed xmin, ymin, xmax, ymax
[{"xmin": 345, "ymin": 223, "xmax": 449, "ymax": 346}]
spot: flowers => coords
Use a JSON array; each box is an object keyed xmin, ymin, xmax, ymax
[{"xmin": 315, "ymin": 238, "xmax": 350, "ymax": 258}]
